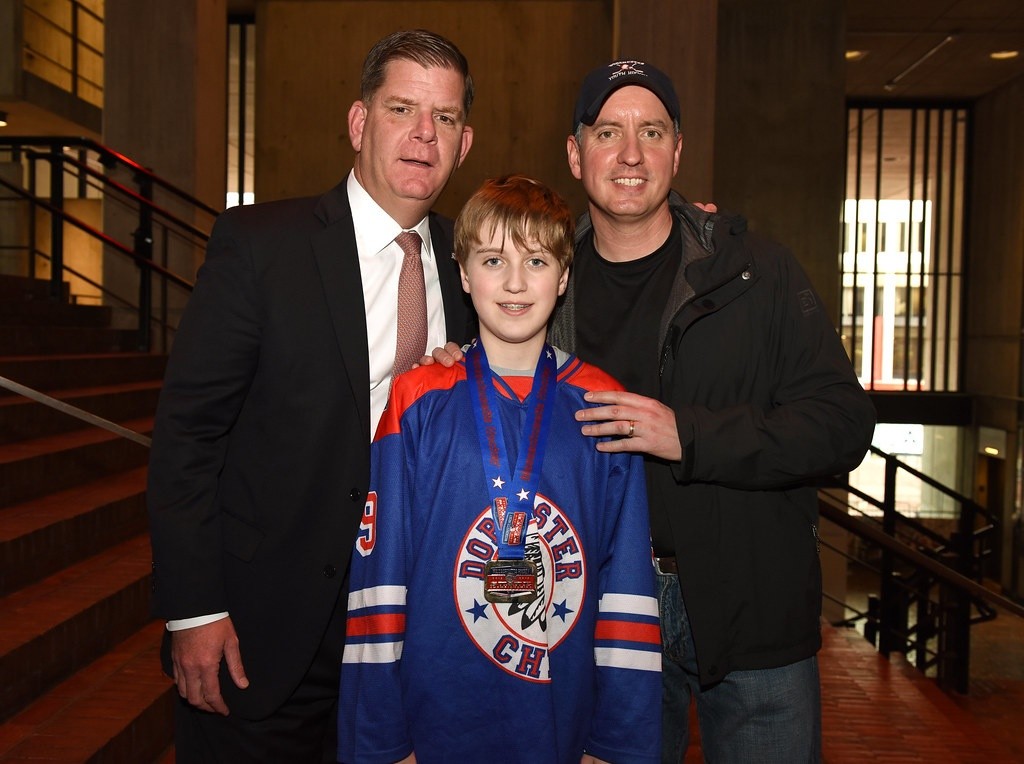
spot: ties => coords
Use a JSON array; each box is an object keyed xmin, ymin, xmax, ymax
[{"xmin": 390, "ymin": 233, "xmax": 429, "ymax": 405}]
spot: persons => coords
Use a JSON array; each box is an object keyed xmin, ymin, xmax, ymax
[
  {"xmin": 143, "ymin": 26, "xmax": 476, "ymax": 764},
  {"xmin": 335, "ymin": 173, "xmax": 664, "ymax": 764},
  {"xmin": 409, "ymin": 58, "xmax": 879, "ymax": 764}
]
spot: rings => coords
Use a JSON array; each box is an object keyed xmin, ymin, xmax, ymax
[{"xmin": 627, "ymin": 420, "xmax": 635, "ymax": 438}]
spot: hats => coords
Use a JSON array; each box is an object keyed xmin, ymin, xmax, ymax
[{"xmin": 574, "ymin": 58, "xmax": 680, "ymax": 137}]
